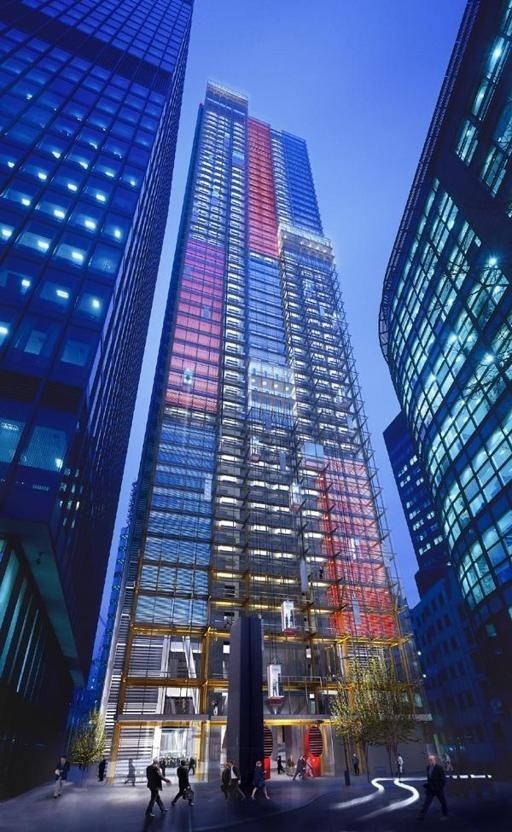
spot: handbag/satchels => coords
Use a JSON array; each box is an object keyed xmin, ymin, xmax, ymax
[{"xmin": 183, "ymin": 785, "xmax": 194, "ymax": 800}]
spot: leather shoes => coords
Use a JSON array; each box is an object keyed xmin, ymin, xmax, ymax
[
  {"xmin": 160, "ymin": 807, "xmax": 168, "ymax": 810},
  {"xmin": 145, "ymin": 811, "xmax": 156, "ymax": 817}
]
